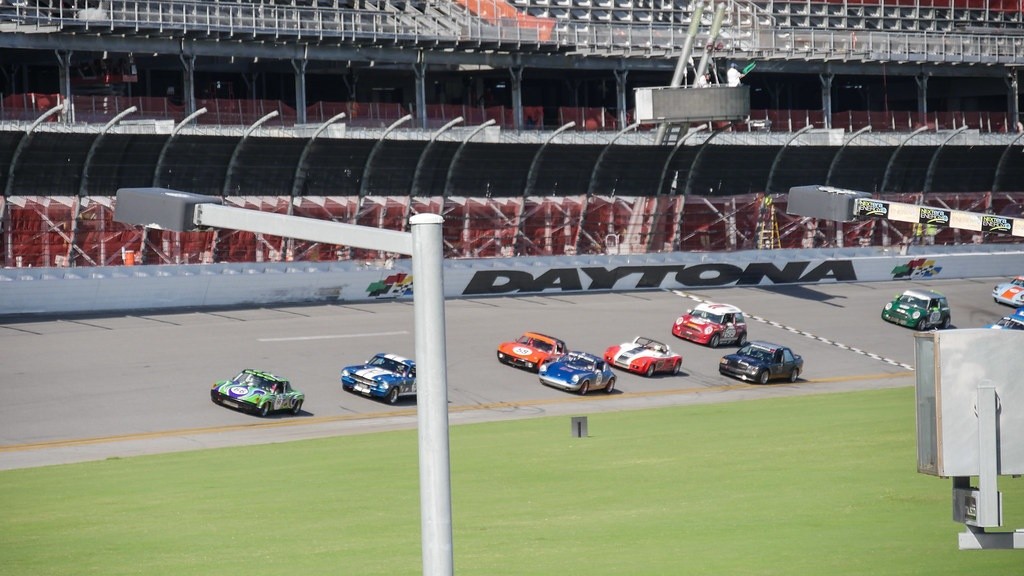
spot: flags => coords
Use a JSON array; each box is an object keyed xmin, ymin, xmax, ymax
[{"xmin": 743, "ymin": 63, "xmax": 755, "ymax": 73}]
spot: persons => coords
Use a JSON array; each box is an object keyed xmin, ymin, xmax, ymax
[
  {"xmin": 268, "ymin": 383, "xmax": 279, "ymax": 394},
  {"xmin": 727, "ymin": 63, "xmax": 745, "ymax": 86},
  {"xmin": 347, "ymin": 95, "xmax": 358, "ymax": 117},
  {"xmin": 398, "ymin": 365, "xmax": 412, "ymax": 377},
  {"xmin": 698, "ymin": 73, "xmax": 712, "ymax": 89},
  {"xmin": 654, "ymin": 345, "xmax": 661, "ymax": 352}
]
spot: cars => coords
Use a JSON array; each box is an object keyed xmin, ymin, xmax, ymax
[
  {"xmin": 882, "ymin": 288, "xmax": 951, "ymax": 331},
  {"xmin": 605, "ymin": 337, "xmax": 682, "ymax": 378},
  {"xmin": 342, "ymin": 353, "xmax": 417, "ymax": 404},
  {"xmin": 672, "ymin": 303, "xmax": 748, "ymax": 349},
  {"xmin": 497, "ymin": 332, "xmax": 567, "ymax": 372},
  {"xmin": 719, "ymin": 340, "xmax": 803, "ymax": 385},
  {"xmin": 990, "ymin": 276, "xmax": 1024, "ymax": 309},
  {"xmin": 211, "ymin": 368, "xmax": 305, "ymax": 418},
  {"xmin": 989, "ymin": 308, "xmax": 1024, "ymax": 331},
  {"xmin": 538, "ymin": 350, "xmax": 617, "ymax": 396}
]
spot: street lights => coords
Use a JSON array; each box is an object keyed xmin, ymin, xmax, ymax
[{"xmin": 110, "ymin": 188, "xmax": 452, "ymax": 576}]
